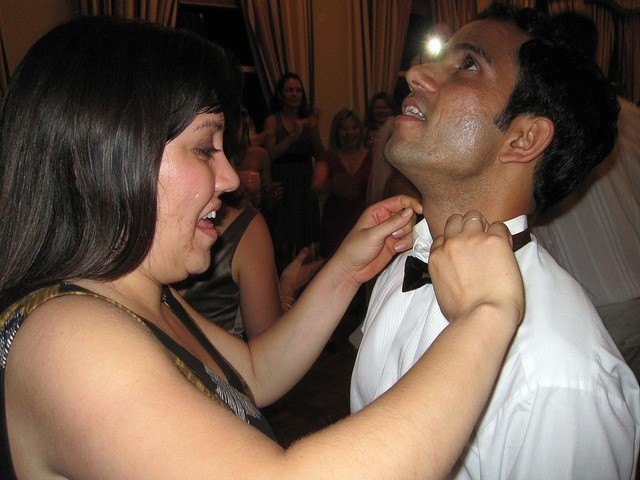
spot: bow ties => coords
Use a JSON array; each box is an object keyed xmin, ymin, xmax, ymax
[{"xmin": 402, "ymin": 228, "xmax": 532, "ymax": 292}]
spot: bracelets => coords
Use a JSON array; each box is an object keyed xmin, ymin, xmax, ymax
[{"xmin": 278, "ymin": 296, "xmax": 296, "ymax": 312}]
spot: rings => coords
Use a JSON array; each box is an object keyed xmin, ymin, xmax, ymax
[
  {"xmin": 272, "ymin": 191, "xmax": 279, "ymax": 197},
  {"xmin": 464, "ymin": 217, "xmax": 484, "ymax": 225}
]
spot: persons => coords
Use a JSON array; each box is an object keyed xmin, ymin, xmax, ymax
[
  {"xmin": 163, "ymin": 205, "xmax": 328, "ymax": 341},
  {"xmin": 312, "ymin": 108, "xmax": 372, "ymax": 256},
  {"xmin": 369, "ymin": 91, "xmax": 391, "ymax": 126},
  {"xmin": 1, "ymin": 17, "xmax": 526, "ymax": 480},
  {"xmin": 348, "ymin": 0, "xmax": 640, "ymax": 480},
  {"xmin": 264, "ymin": 71, "xmax": 324, "ymax": 253},
  {"xmin": 226, "ymin": 108, "xmax": 284, "ymax": 202}
]
